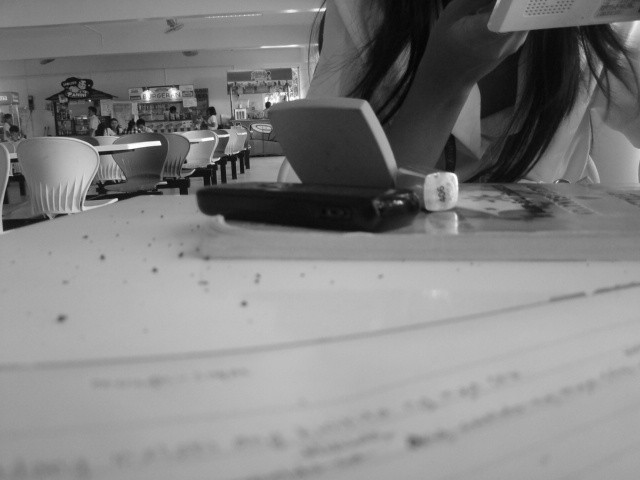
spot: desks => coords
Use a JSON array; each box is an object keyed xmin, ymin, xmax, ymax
[
  {"xmin": 9, "ymin": 140, "xmax": 163, "ymax": 194},
  {"xmin": 186, "ymin": 137, "xmax": 216, "ymax": 143},
  {"xmin": 1, "ymin": 182, "xmax": 640, "ymax": 480}
]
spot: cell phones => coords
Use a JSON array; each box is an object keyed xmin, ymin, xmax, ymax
[{"xmin": 197, "ymin": 182, "xmax": 422, "ymax": 233}]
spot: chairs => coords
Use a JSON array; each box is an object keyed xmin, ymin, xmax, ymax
[
  {"xmin": 95, "ymin": 135, "xmax": 126, "ymax": 182},
  {"xmin": 225, "ymin": 128, "xmax": 240, "ymax": 179},
  {"xmin": 158, "ymin": 133, "xmax": 196, "ymax": 194},
  {"xmin": 241, "ymin": 125, "xmax": 252, "ymax": 169},
  {"xmin": 0, "ymin": 143, "xmax": 11, "ymax": 232},
  {"xmin": 74, "ymin": 135, "xmax": 100, "ymax": 146},
  {"xmin": 213, "ymin": 129, "xmax": 231, "ymax": 184},
  {"xmin": 232, "ymin": 126, "xmax": 247, "ymax": 174},
  {"xmin": 15, "ymin": 136, "xmax": 119, "ymax": 221},
  {"xmin": 181, "ymin": 130, "xmax": 219, "ymax": 186},
  {"xmin": 104, "ymin": 132, "xmax": 169, "ymax": 195}
]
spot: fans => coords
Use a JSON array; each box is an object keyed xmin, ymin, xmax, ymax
[{"xmin": 162, "ymin": 18, "xmax": 184, "ymax": 35}]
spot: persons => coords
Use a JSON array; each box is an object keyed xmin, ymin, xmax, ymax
[
  {"xmin": 3, "ymin": 113, "xmax": 14, "ymax": 142},
  {"xmin": 137, "ymin": 118, "xmax": 153, "ymax": 134},
  {"xmin": 122, "ymin": 120, "xmax": 142, "ymax": 134},
  {"xmin": 103, "ymin": 118, "xmax": 120, "ymax": 135},
  {"xmin": 87, "ymin": 106, "xmax": 99, "ymax": 136},
  {"xmin": 264, "ymin": 102, "xmax": 272, "ymax": 118},
  {"xmin": 303, "ymin": 2, "xmax": 640, "ymax": 184},
  {"xmin": 203, "ymin": 106, "xmax": 218, "ymax": 130},
  {"xmin": 10, "ymin": 124, "xmax": 20, "ymax": 140},
  {"xmin": 164, "ymin": 106, "xmax": 180, "ymax": 120}
]
